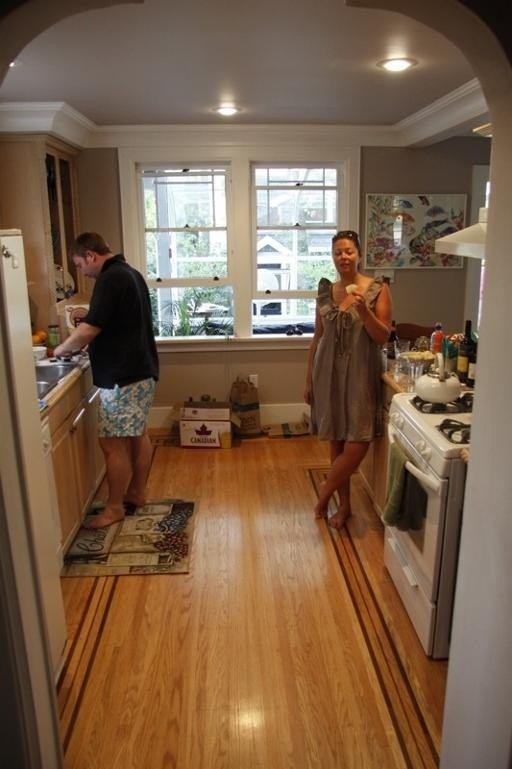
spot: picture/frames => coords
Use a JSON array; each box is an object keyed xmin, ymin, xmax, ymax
[{"xmin": 361, "ymin": 191, "xmax": 468, "ymax": 273}]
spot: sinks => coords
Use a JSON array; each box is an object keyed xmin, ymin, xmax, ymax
[
  {"xmin": 37, "ymin": 382, "xmax": 51, "ymax": 399},
  {"xmin": 35, "ymin": 363, "xmax": 78, "ymax": 379}
]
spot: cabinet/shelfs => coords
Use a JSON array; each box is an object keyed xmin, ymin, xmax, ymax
[
  {"xmin": 70, "ymin": 368, "xmax": 116, "ymax": 521},
  {"xmin": 0, "ymin": 126, "xmax": 89, "ymax": 341},
  {"xmin": 43, "ymin": 386, "xmax": 87, "ymax": 556},
  {"xmin": 358, "ymin": 432, "xmax": 374, "ymax": 498},
  {"xmin": 374, "ymin": 369, "xmax": 409, "ymax": 536}
]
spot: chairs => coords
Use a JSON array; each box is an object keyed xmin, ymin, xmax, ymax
[{"xmin": 394, "ymin": 321, "xmax": 436, "ymax": 350}]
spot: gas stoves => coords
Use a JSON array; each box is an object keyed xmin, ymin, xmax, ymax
[{"xmin": 392, "ymin": 389, "xmax": 475, "ymax": 478}]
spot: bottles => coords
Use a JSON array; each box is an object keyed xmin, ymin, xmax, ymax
[
  {"xmin": 430, "ymin": 321, "xmax": 445, "ymax": 351},
  {"xmin": 466, "ymin": 340, "xmax": 479, "ymax": 387},
  {"xmin": 386, "ymin": 319, "xmax": 400, "ymax": 361},
  {"xmin": 47, "ymin": 324, "xmax": 60, "ymax": 347},
  {"xmin": 457, "ymin": 319, "xmax": 474, "ymax": 383}
]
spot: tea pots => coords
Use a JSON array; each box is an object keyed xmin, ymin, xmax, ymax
[{"xmin": 414, "ymin": 352, "xmax": 463, "ymax": 406}]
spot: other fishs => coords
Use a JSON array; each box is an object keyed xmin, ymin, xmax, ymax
[
  {"xmin": 426, "ymin": 217, "xmax": 451, "ymax": 229},
  {"xmin": 372, "ymin": 237, "xmax": 394, "ymax": 248},
  {"xmin": 426, "ymin": 206, "xmax": 448, "ymax": 216},
  {"xmin": 393, "ymin": 200, "xmax": 413, "ymax": 208},
  {"xmin": 418, "ymin": 195, "xmax": 429, "ymax": 206},
  {"xmin": 387, "ymin": 212, "xmax": 415, "ymax": 223}
]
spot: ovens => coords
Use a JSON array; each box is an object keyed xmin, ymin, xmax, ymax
[{"xmin": 380, "ymin": 425, "xmax": 448, "ymax": 604}]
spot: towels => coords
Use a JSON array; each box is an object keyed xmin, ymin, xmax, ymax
[{"xmin": 379, "ymin": 439, "xmax": 417, "ymax": 532}]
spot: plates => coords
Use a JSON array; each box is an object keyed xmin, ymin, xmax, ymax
[{"xmin": 52, "ymin": 265, "xmax": 76, "ymax": 299}]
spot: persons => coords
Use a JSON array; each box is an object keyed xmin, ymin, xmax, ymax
[
  {"xmin": 50, "ymin": 231, "xmax": 158, "ymax": 531},
  {"xmin": 303, "ymin": 230, "xmax": 394, "ymax": 529}
]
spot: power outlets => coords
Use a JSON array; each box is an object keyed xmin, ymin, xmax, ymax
[{"xmin": 248, "ymin": 373, "xmax": 258, "ymax": 389}]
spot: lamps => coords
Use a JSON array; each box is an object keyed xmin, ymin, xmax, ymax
[{"xmin": 434, "ymin": 202, "xmax": 490, "ymax": 260}]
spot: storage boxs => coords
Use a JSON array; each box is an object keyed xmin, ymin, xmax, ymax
[
  {"xmin": 234, "ymin": 401, "xmax": 262, "ymax": 438},
  {"xmin": 167, "ymin": 400, "xmax": 240, "ymax": 449}
]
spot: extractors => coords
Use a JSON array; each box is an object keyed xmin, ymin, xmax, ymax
[{"xmin": 435, "ymin": 207, "xmax": 488, "ymax": 259}]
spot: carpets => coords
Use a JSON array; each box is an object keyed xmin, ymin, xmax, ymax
[{"xmin": 61, "ymin": 493, "xmax": 200, "ymax": 579}]
